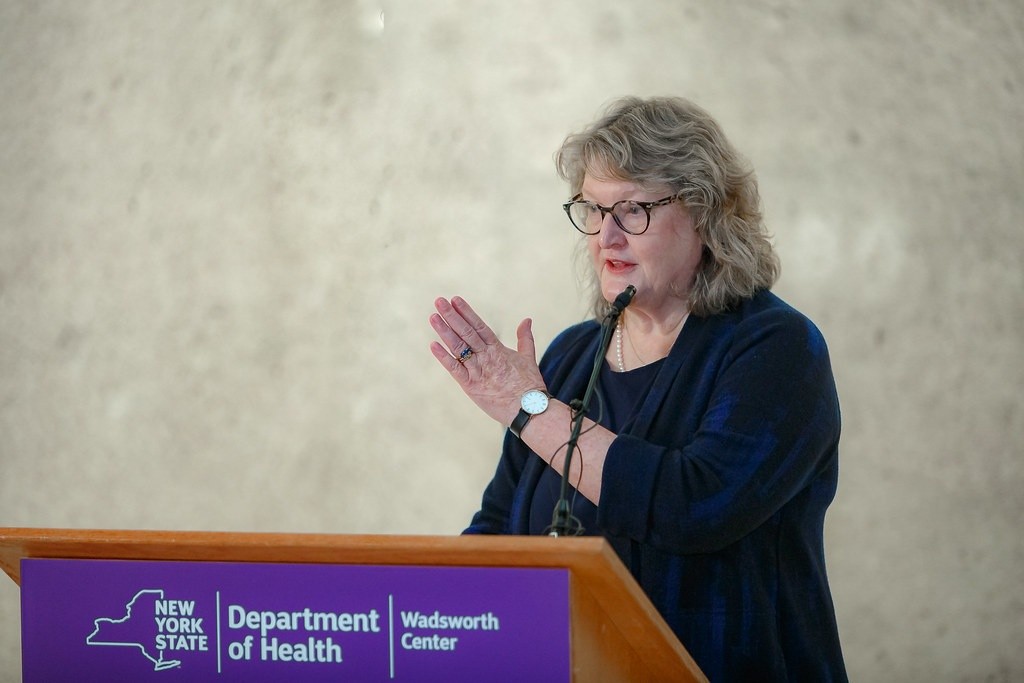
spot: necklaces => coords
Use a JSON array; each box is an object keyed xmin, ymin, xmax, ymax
[{"xmin": 617, "ymin": 320, "xmax": 625, "ymax": 372}]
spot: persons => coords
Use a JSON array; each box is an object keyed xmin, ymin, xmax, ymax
[{"xmin": 431, "ymin": 95, "xmax": 848, "ymax": 683}]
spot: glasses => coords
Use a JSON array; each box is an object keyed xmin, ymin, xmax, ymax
[{"xmin": 561, "ymin": 191, "xmax": 683, "ymax": 235}]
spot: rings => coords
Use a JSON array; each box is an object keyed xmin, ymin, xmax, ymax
[{"xmin": 458, "ymin": 346, "xmax": 472, "ymax": 363}]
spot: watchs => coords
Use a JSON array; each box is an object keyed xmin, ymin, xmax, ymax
[{"xmin": 509, "ymin": 389, "xmax": 555, "ymax": 438}]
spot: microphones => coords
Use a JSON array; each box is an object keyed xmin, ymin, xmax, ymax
[{"xmin": 539, "ymin": 284, "xmax": 637, "ymax": 537}]
